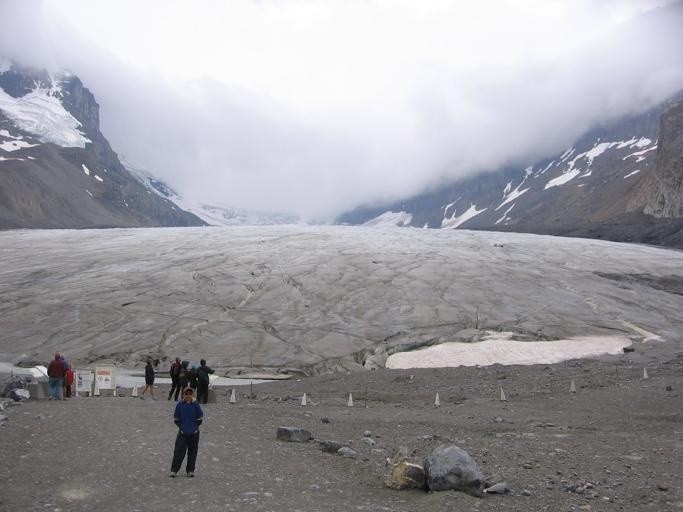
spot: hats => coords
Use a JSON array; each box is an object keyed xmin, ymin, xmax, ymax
[{"xmin": 183, "ymin": 387, "xmax": 194, "ymax": 392}]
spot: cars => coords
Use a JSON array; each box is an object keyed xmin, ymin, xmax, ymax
[{"xmin": 11, "ymin": 359, "xmax": 49, "ymax": 381}]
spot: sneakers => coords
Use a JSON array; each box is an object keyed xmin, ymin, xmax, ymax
[
  {"xmin": 187, "ymin": 472, "xmax": 194, "ymax": 477},
  {"xmin": 169, "ymin": 472, "xmax": 177, "ymax": 477}
]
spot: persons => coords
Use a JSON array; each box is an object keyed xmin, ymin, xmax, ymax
[
  {"xmin": 193, "ymin": 357, "xmax": 215, "ymax": 404},
  {"xmin": 186, "ymin": 365, "xmax": 196, "ymax": 394},
  {"xmin": 57, "ymin": 354, "xmax": 68, "ymax": 396},
  {"xmin": 175, "ymin": 360, "xmax": 189, "ymax": 399},
  {"xmin": 168, "ymin": 387, "xmax": 203, "ymax": 477},
  {"xmin": 60, "ymin": 367, "xmax": 73, "ymax": 399},
  {"xmin": 139, "ymin": 356, "xmax": 156, "ymax": 399},
  {"xmin": 167, "ymin": 356, "xmax": 180, "ymax": 401},
  {"xmin": 44, "ymin": 352, "xmax": 62, "ymax": 400}
]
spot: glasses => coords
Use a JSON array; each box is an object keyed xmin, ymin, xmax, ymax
[{"xmin": 185, "ymin": 393, "xmax": 192, "ymax": 395}]
